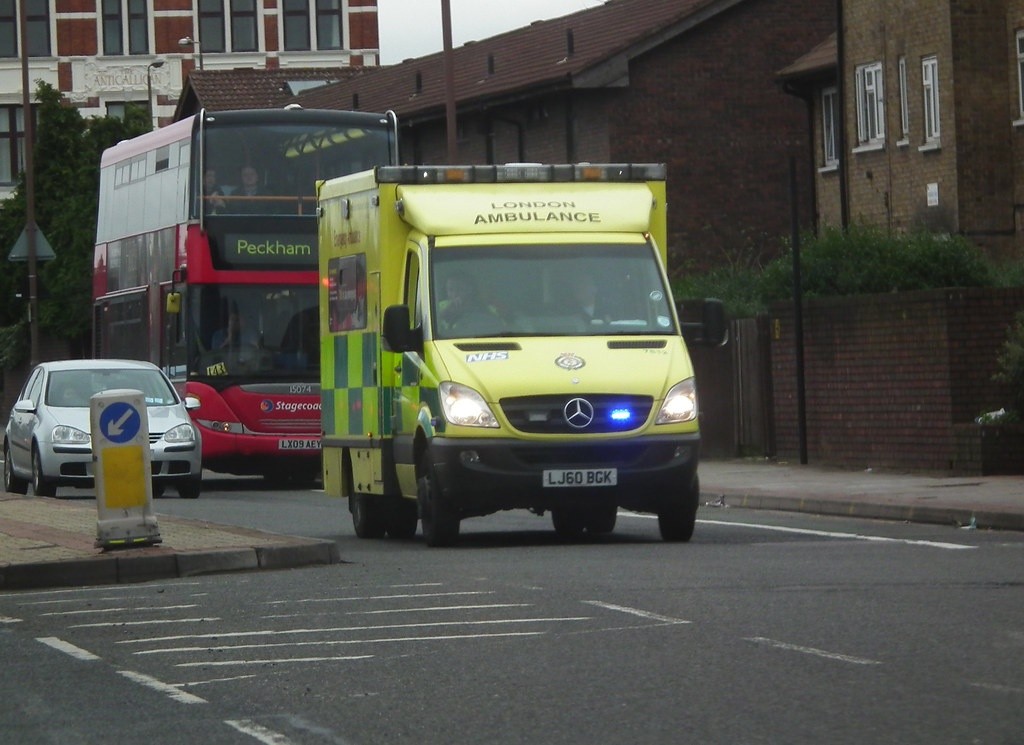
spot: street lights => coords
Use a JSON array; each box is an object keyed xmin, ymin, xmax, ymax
[
  {"xmin": 147, "ymin": 59, "xmax": 165, "ymax": 133},
  {"xmin": 177, "ymin": 37, "xmax": 204, "ymax": 70}
]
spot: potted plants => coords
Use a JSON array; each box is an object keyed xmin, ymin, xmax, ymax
[{"xmin": 979, "ymin": 409, "xmax": 1024, "ymax": 476}]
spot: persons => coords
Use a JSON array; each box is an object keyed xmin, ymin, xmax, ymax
[
  {"xmin": 438, "ymin": 271, "xmax": 514, "ymax": 339},
  {"xmin": 555, "ymin": 268, "xmax": 620, "ymax": 333},
  {"xmin": 211, "ymin": 310, "xmax": 265, "ymax": 354},
  {"xmin": 203, "ymin": 163, "xmax": 267, "ymax": 216}
]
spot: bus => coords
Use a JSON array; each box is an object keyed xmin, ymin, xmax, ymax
[{"xmin": 92, "ymin": 103, "xmax": 400, "ymax": 488}]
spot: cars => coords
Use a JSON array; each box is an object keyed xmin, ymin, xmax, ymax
[{"xmin": 2, "ymin": 359, "xmax": 205, "ymax": 501}]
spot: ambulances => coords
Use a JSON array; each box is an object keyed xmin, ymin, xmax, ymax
[{"xmin": 314, "ymin": 161, "xmax": 706, "ymax": 543}]
